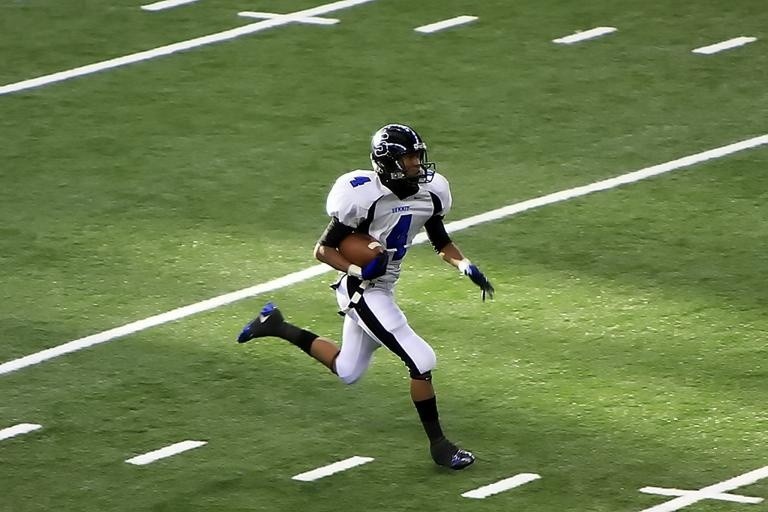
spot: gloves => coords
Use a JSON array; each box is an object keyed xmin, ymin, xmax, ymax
[
  {"xmin": 361, "ymin": 249, "xmax": 388, "ymax": 280},
  {"xmin": 464, "ymin": 265, "xmax": 495, "ymax": 300}
]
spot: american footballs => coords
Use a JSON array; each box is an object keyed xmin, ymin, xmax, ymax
[{"xmin": 338, "ymin": 234, "xmax": 386, "ymax": 266}]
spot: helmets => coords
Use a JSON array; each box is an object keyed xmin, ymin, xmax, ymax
[{"xmin": 370, "ymin": 124, "xmax": 435, "ymax": 199}]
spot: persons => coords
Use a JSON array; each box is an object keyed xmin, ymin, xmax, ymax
[{"xmin": 237, "ymin": 124, "xmax": 494, "ymax": 469}]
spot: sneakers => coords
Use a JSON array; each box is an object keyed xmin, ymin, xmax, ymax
[
  {"xmin": 238, "ymin": 303, "xmax": 283, "ymax": 343},
  {"xmin": 430, "ymin": 441, "xmax": 475, "ymax": 470}
]
